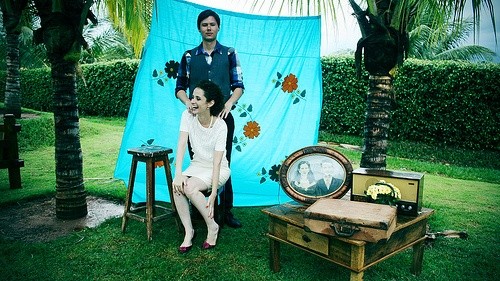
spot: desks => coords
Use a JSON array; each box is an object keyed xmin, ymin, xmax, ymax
[{"xmin": 262, "ymin": 193, "xmax": 436, "ymax": 281}]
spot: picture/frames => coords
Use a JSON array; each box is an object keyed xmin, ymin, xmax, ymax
[{"xmin": 280, "ymin": 146, "xmax": 354, "ymax": 203}]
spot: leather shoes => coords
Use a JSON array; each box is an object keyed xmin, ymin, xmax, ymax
[
  {"xmin": 224, "ymin": 213, "xmax": 244, "ymax": 227},
  {"xmin": 216, "ymin": 212, "xmax": 224, "ymax": 226}
]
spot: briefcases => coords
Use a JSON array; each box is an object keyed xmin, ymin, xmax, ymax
[{"xmin": 304, "ymin": 198, "xmax": 397, "ymax": 245}]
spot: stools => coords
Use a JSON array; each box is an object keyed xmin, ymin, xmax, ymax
[
  {"xmin": 121, "ymin": 145, "xmax": 185, "ymax": 241},
  {"xmin": 191, "ymin": 185, "xmax": 226, "ymax": 232}
]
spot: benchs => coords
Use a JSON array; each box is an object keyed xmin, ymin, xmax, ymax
[{"xmin": 0, "ymin": 114, "xmax": 24, "ymax": 189}]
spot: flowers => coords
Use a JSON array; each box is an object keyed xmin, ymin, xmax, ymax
[{"xmin": 365, "ymin": 180, "xmax": 402, "ymax": 206}]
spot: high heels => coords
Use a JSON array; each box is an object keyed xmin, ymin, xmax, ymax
[
  {"xmin": 179, "ymin": 230, "xmax": 196, "ymax": 252},
  {"xmin": 202, "ymin": 226, "xmax": 220, "ymax": 249}
]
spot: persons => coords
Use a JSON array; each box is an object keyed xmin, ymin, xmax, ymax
[
  {"xmin": 172, "ymin": 80, "xmax": 231, "ymax": 252},
  {"xmin": 175, "ymin": 9, "xmax": 244, "ymax": 228},
  {"xmin": 289, "ymin": 159, "xmax": 343, "ymax": 197}
]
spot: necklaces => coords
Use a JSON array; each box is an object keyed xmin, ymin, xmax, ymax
[{"xmin": 208, "ymin": 116, "xmax": 214, "ymax": 128}]
